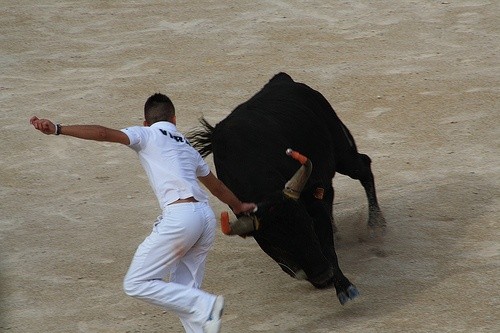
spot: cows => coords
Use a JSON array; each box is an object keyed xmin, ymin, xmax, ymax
[{"xmin": 183, "ymin": 70, "xmax": 388, "ymax": 306}]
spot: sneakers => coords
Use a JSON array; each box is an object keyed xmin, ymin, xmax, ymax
[{"xmin": 202, "ymin": 295, "xmax": 223, "ymax": 333}]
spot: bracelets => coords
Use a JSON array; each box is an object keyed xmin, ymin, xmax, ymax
[{"xmin": 55, "ymin": 123, "xmax": 62, "ymax": 136}]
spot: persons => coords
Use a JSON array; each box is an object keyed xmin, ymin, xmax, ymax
[{"xmin": 28, "ymin": 92, "xmax": 257, "ymax": 333}]
